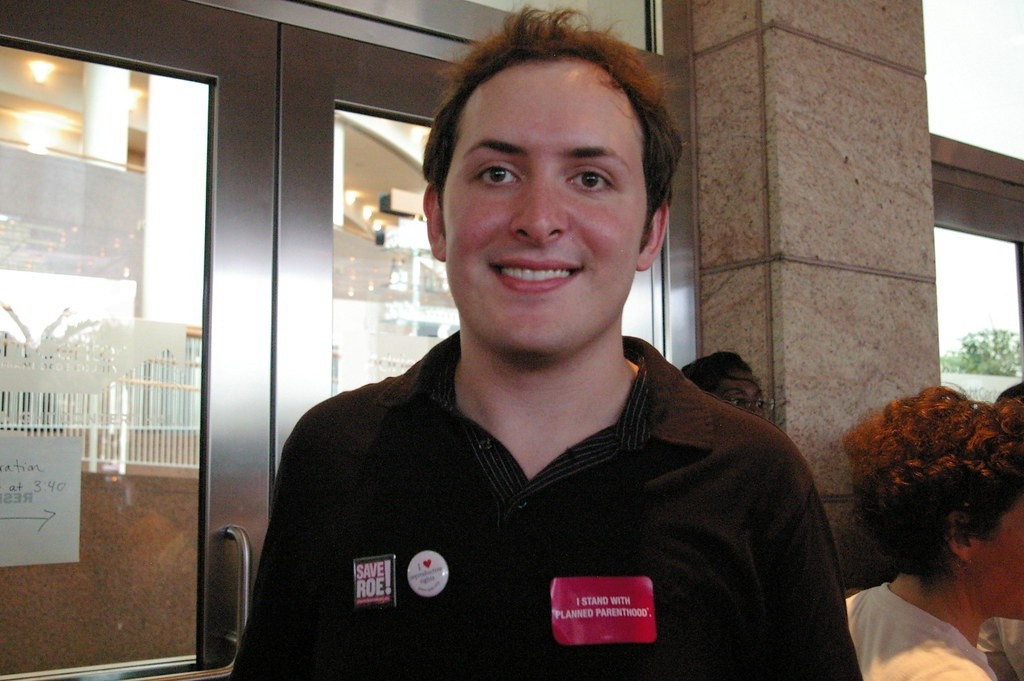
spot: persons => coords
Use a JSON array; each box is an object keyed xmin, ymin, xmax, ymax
[
  {"xmin": 679, "ymin": 352, "xmax": 1024, "ymax": 680},
  {"xmin": 229, "ymin": 8, "xmax": 866, "ymax": 680}
]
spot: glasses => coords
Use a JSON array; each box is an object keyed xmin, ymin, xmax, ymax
[{"xmin": 718, "ymin": 387, "xmax": 776, "ymax": 413}]
